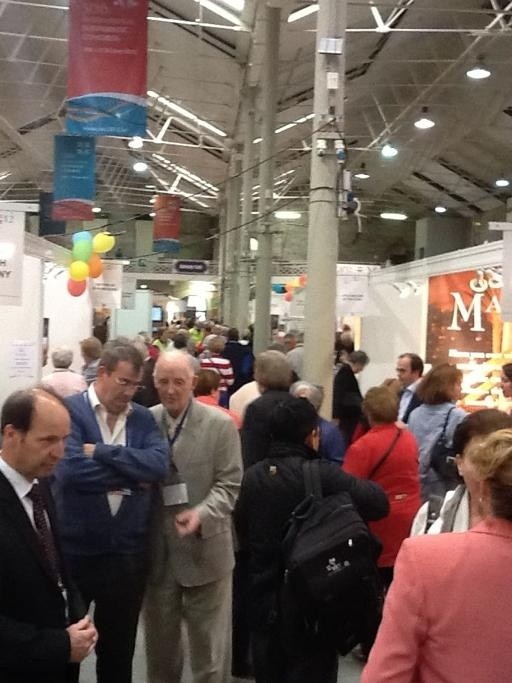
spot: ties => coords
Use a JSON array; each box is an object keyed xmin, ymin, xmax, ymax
[{"xmin": 27, "ymin": 484, "xmax": 66, "ymax": 591}]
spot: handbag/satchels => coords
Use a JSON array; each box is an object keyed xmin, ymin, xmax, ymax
[{"xmin": 431, "ymin": 439, "xmax": 458, "ymax": 479}]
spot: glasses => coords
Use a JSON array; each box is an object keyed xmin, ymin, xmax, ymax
[{"xmin": 114, "ymin": 378, "xmax": 146, "ymax": 393}]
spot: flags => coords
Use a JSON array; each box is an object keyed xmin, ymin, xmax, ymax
[
  {"xmin": 53, "ymin": 136, "xmax": 95, "ymax": 222},
  {"xmin": 153, "ymin": 194, "xmax": 180, "ymax": 252},
  {"xmin": 39, "ymin": 188, "xmax": 66, "ymax": 237},
  {"xmin": 61, "ymin": 0, "xmax": 147, "ymax": 138}
]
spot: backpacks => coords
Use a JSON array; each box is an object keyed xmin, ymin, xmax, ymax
[{"xmin": 275, "ymin": 460, "xmax": 385, "ymax": 657}]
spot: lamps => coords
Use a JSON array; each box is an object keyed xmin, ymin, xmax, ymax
[{"xmin": 354, "ymin": 52, "xmax": 492, "ymax": 180}]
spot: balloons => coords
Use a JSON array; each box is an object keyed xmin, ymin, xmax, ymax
[
  {"xmin": 67, "ymin": 277, "xmax": 86, "ymax": 297},
  {"xmin": 86, "ymin": 253, "xmax": 104, "ymax": 278},
  {"xmin": 271, "ymin": 274, "xmax": 309, "ymax": 302},
  {"xmin": 71, "ymin": 239, "xmax": 92, "ymax": 262},
  {"xmin": 71, "ymin": 230, "xmax": 92, "ymax": 247},
  {"xmin": 92, "ymin": 231, "xmax": 116, "ymax": 254},
  {"xmin": 69, "ymin": 261, "xmax": 89, "ymax": 282}
]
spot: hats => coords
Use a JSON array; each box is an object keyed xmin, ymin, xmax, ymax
[{"xmin": 271, "ymin": 398, "xmax": 317, "ymax": 438}]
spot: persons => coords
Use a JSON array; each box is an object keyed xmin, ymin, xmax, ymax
[{"xmin": 1, "ymin": 307, "xmax": 511, "ymax": 683}]
